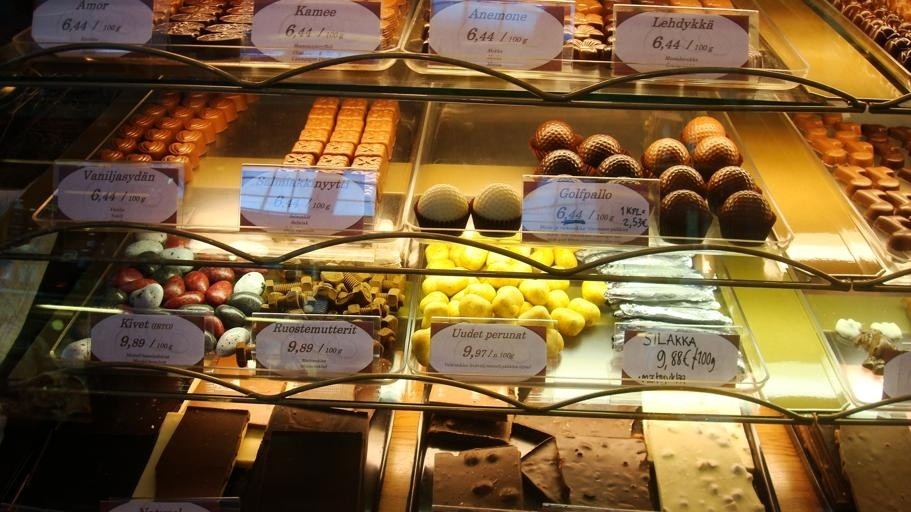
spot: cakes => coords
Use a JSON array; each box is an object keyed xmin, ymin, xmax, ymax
[
  {"xmin": 235, "ymin": 248, "xmax": 418, "ymax": 372},
  {"xmin": 641, "ymin": 394, "xmax": 911, "ymax": 512},
  {"xmin": 369, "ymin": 2, "xmax": 409, "ymax": 54},
  {"xmin": 547, "ymin": 174, "xmax": 580, "ymax": 187},
  {"xmin": 526, "ymin": 118, "xmax": 577, "ymax": 160},
  {"xmin": 574, "ymin": 246, "xmax": 746, "ymax": 392},
  {"xmin": 679, "ymin": 114, "xmax": 727, "ymax": 148},
  {"xmin": 564, "ymin": 1, "xmax": 740, "ymax": 79},
  {"xmin": 55, "ymin": 228, "xmax": 269, "ymax": 374},
  {"xmin": 606, "ymin": 174, "xmax": 658, "ymax": 217},
  {"xmin": 412, "ymin": 237, "xmax": 610, "ymax": 381},
  {"xmin": 718, "ymin": 189, "xmax": 778, "ymax": 248},
  {"xmin": 692, "ymin": 134, "xmax": 745, "ymax": 180},
  {"xmin": 279, "ymin": 91, "xmax": 400, "ymax": 247},
  {"xmin": 99, "ymin": 81, "xmax": 261, "ymax": 221},
  {"xmin": 145, "ymin": 1, "xmax": 257, "ymax": 64},
  {"xmin": 411, "ymin": 183, "xmax": 471, "ymax": 241},
  {"xmin": 832, "ymin": 293, "xmax": 910, "ymax": 418},
  {"xmin": 654, "ymin": 187, "xmax": 713, "ymax": 247},
  {"xmin": 471, "ymin": 183, "xmax": 524, "ymax": 239},
  {"xmin": 414, "ymin": 378, "xmax": 654, "ymax": 512},
  {"xmin": 591, "ymin": 152, "xmax": 643, "ymax": 183},
  {"xmin": 706, "ymin": 165, "xmax": 765, "ymax": 219},
  {"xmin": 641, "ymin": 137, "xmax": 693, "ymax": 178},
  {"xmin": 781, "ymin": 104, "xmax": 910, "ymax": 284},
  {"xmin": 532, "ymin": 147, "xmax": 585, "ymax": 178},
  {"xmin": 573, "ymin": 132, "xmax": 624, "ymax": 168},
  {"xmin": 655, "ymin": 164, "xmax": 707, "ymax": 203}
]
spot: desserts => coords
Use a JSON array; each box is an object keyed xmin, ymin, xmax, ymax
[
  {"xmin": 154, "ymin": 405, "xmax": 250, "ymax": 504},
  {"xmin": 246, "ymin": 401, "xmax": 369, "ymax": 510}
]
